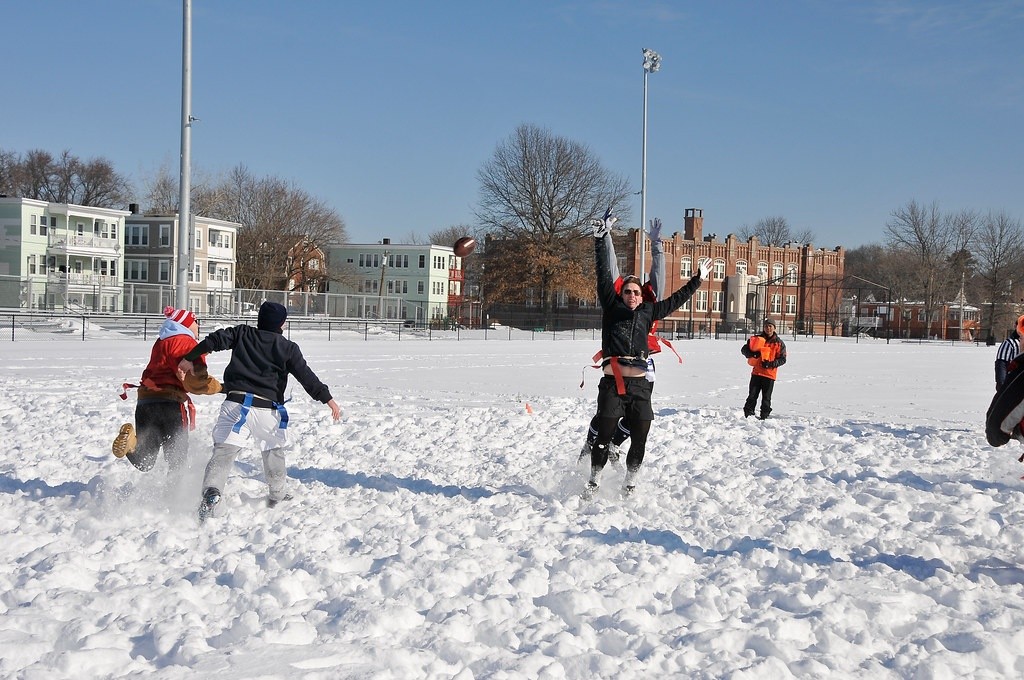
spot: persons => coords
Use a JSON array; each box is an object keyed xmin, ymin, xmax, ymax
[
  {"xmin": 178, "ymin": 300, "xmax": 340, "ymax": 521},
  {"xmin": 577, "ymin": 207, "xmax": 665, "ymax": 470},
  {"xmin": 985, "ymin": 315, "xmax": 1024, "ymax": 469},
  {"xmin": 112, "ymin": 305, "xmax": 227, "ymax": 504},
  {"xmin": 587, "ymin": 219, "xmax": 714, "ymax": 492},
  {"xmin": 741, "ymin": 319, "xmax": 786, "ymax": 420},
  {"xmin": 995, "ymin": 320, "xmax": 1021, "ymax": 391}
]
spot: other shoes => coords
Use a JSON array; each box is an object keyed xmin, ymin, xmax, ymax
[
  {"xmin": 608, "ymin": 443, "xmax": 627, "ymax": 472},
  {"xmin": 112, "ymin": 422, "xmax": 138, "ymax": 458},
  {"xmin": 621, "ymin": 482, "xmax": 635, "ymax": 501},
  {"xmin": 193, "ymin": 487, "xmax": 221, "ymax": 522},
  {"xmin": 270, "ymin": 494, "xmax": 294, "ymax": 508},
  {"xmin": 574, "ymin": 443, "xmax": 597, "ymax": 468},
  {"xmin": 582, "ymin": 480, "xmax": 600, "ymax": 500}
]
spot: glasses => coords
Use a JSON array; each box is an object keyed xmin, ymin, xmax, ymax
[
  {"xmin": 624, "ymin": 289, "xmax": 640, "ymax": 296},
  {"xmin": 194, "ymin": 319, "xmax": 198, "ymax": 324}
]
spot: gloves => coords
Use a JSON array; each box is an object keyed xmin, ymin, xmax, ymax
[
  {"xmin": 590, "ymin": 219, "xmax": 610, "ymax": 239},
  {"xmin": 603, "ymin": 206, "xmax": 617, "ymax": 230},
  {"xmin": 698, "ymin": 257, "xmax": 715, "ymax": 280},
  {"xmin": 749, "ymin": 352, "xmax": 761, "ymax": 359},
  {"xmin": 218, "ymin": 384, "xmax": 227, "ymax": 393},
  {"xmin": 642, "ymin": 217, "xmax": 662, "ymax": 241},
  {"xmin": 762, "ymin": 358, "xmax": 772, "ymax": 369}
]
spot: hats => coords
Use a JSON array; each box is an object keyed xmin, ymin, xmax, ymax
[
  {"xmin": 763, "ymin": 319, "xmax": 777, "ymax": 330},
  {"xmin": 258, "ymin": 301, "xmax": 288, "ymax": 330},
  {"xmin": 164, "ymin": 306, "xmax": 196, "ymax": 329}
]
